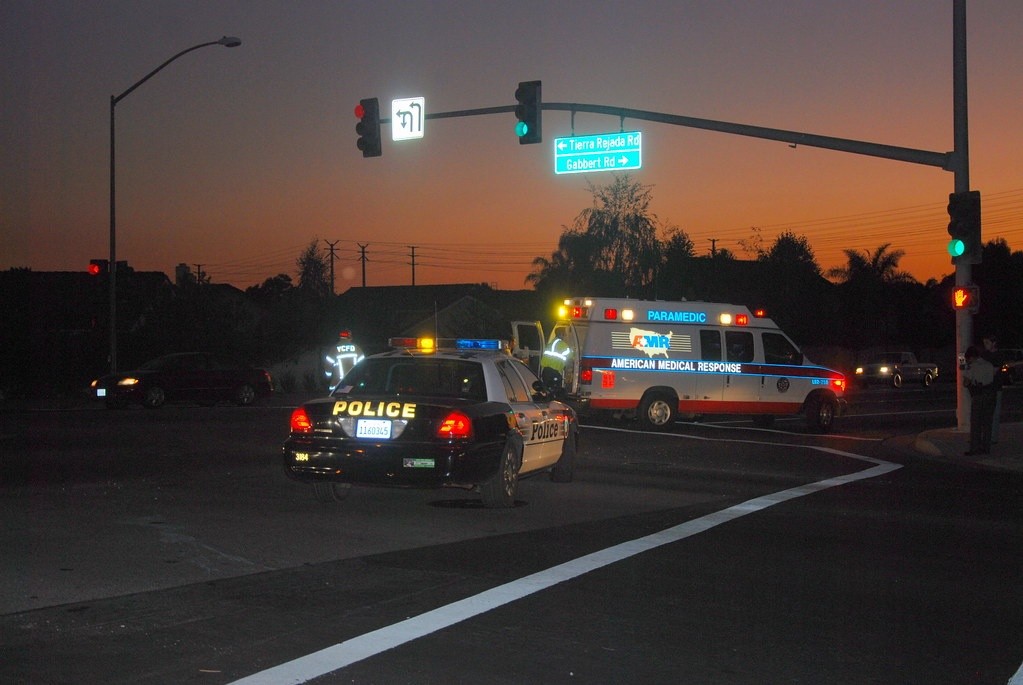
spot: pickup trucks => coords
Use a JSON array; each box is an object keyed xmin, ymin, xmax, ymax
[{"xmin": 855, "ymin": 350, "xmax": 939, "ymax": 386}]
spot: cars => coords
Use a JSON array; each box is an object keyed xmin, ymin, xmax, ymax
[{"xmin": 283, "ymin": 337, "xmax": 580, "ymax": 507}]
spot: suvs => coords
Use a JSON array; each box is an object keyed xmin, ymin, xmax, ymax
[{"xmin": 90, "ymin": 352, "xmax": 273, "ymax": 410}]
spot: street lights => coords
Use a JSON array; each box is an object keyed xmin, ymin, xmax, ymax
[{"xmin": 110, "ymin": 36, "xmax": 242, "ymax": 375}]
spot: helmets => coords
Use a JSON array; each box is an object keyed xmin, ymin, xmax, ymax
[{"xmin": 339, "ymin": 329, "xmax": 352, "ymax": 340}]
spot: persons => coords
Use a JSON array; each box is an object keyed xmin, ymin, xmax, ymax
[
  {"xmin": 541, "ymin": 327, "xmax": 573, "ymax": 385},
  {"xmin": 325, "ymin": 329, "xmax": 365, "ymax": 391},
  {"xmin": 964, "ymin": 346, "xmax": 993, "ymax": 456},
  {"xmin": 502, "ymin": 336, "xmax": 527, "ymax": 364},
  {"xmin": 981, "ymin": 335, "xmax": 1002, "ymax": 445},
  {"xmin": 835, "ymin": 342, "xmax": 856, "ymax": 369}
]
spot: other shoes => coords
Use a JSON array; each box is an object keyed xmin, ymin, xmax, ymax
[
  {"xmin": 990, "ymin": 439, "xmax": 997, "ymax": 444},
  {"xmin": 964, "ymin": 447, "xmax": 990, "ymax": 455}
]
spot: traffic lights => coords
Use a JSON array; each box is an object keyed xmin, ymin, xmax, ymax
[
  {"xmin": 947, "ymin": 191, "xmax": 981, "ymax": 264},
  {"xmin": 515, "ymin": 81, "xmax": 543, "ymax": 145},
  {"xmin": 952, "ymin": 287, "xmax": 968, "ymax": 308},
  {"xmin": 355, "ymin": 98, "xmax": 382, "ymax": 158}
]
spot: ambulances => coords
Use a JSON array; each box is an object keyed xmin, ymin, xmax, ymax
[{"xmin": 510, "ymin": 295, "xmax": 848, "ymax": 433}]
